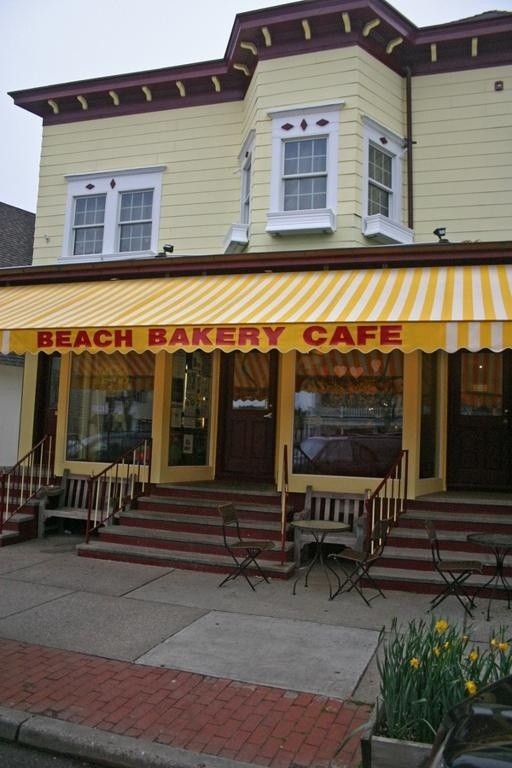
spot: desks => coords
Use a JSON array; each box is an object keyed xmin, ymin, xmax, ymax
[
  {"xmin": 289, "ymin": 519, "xmax": 351, "ymax": 599},
  {"xmin": 467, "ymin": 532, "xmax": 512, "ymax": 621}
]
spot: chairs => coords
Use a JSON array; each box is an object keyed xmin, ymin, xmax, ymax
[
  {"xmin": 327, "ymin": 514, "xmax": 394, "ymax": 608},
  {"xmin": 423, "ymin": 517, "xmax": 483, "ymax": 617},
  {"xmin": 216, "ymin": 501, "xmax": 275, "ymax": 591}
]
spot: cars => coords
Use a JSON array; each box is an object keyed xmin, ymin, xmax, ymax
[
  {"xmin": 296, "ymin": 436, "xmax": 399, "ymax": 477},
  {"xmin": 67, "ymin": 433, "xmax": 149, "ymax": 465}
]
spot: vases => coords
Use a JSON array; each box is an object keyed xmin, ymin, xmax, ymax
[{"xmin": 361, "ymin": 693, "xmax": 433, "ymax": 767}]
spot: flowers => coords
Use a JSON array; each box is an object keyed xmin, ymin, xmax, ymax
[{"xmin": 333, "ymin": 606, "xmax": 512, "ymax": 759}]
